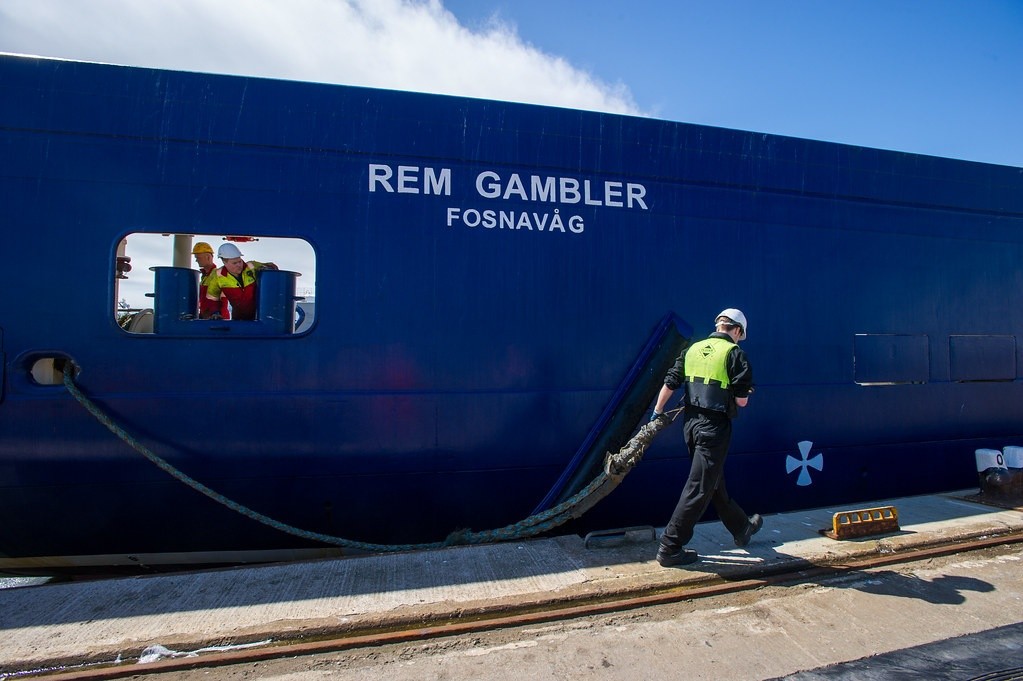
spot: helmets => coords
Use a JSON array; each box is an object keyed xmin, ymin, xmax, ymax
[
  {"xmin": 190, "ymin": 242, "xmax": 214, "ymax": 255},
  {"xmin": 715, "ymin": 308, "xmax": 747, "ymax": 341},
  {"xmin": 217, "ymin": 243, "xmax": 244, "ymax": 259}
]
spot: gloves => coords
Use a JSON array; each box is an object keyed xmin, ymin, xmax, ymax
[{"xmin": 651, "ymin": 409, "xmax": 665, "ymax": 421}]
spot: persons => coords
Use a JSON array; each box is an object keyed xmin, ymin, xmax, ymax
[
  {"xmin": 190, "ymin": 242, "xmax": 230, "ymax": 320},
  {"xmin": 650, "ymin": 308, "xmax": 763, "ymax": 567},
  {"xmin": 205, "ymin": 243, "xmax": 277, "ymax": 320}
]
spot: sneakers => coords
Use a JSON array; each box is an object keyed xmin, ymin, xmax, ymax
[
  {"xmin": 736, "ymin": 514, "xmax": 763, "ymax": 546},
  {"xmin": 657, "ymin": 543, "xmax": 698, "ymax": 566}
]
